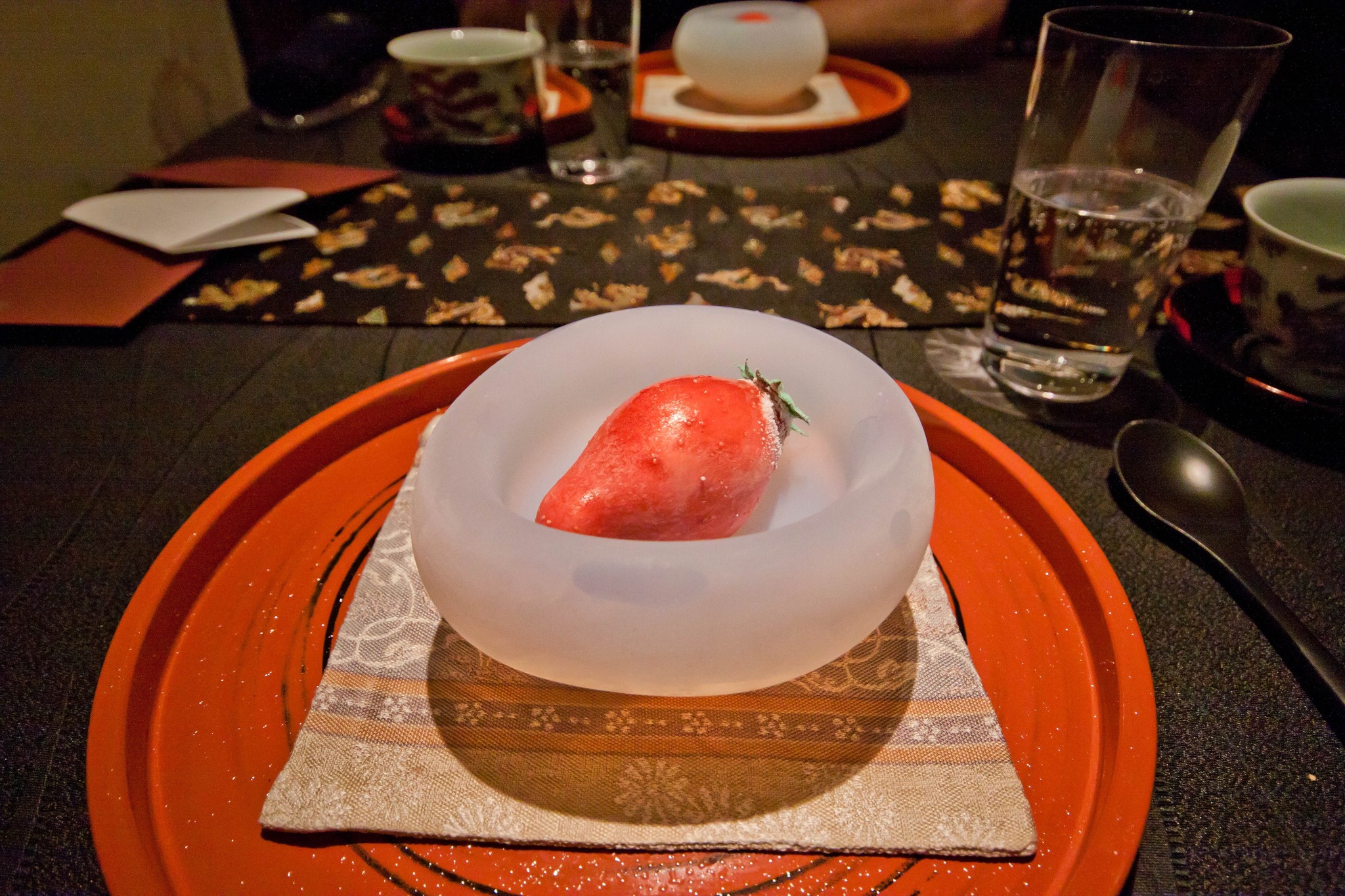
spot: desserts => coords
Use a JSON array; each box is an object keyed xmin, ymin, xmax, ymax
[
  {"xmin": 413, "ymin": 304, "xmax": 933, "ymax": 699},
  {"xmin": 672, "ymin": 0, "xmax": 829, "ymax": 106}
]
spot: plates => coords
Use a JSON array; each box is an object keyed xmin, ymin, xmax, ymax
[
  {"xmin": 387, "ymin": 64, "xmax": 593, "ymax": 146},
  {"xmin": 86, "ymin": 336, "xmax": 1159, "ymax": 896},
  {"xmin": 630, "ymin": 48, "xmax": 910, "ymax": 133}
]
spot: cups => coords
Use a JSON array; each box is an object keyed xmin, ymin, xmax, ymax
[
  {"xmin": 525, "ymin": 0, "xmax": 641, "ymax": 185},
  {"xmin": 1242, "ymin": 177, "xmax": 1345, "ymax": 403},
  {"xmin": 387, "ymin": 27, "xmax": 545, "ymax": 146},
  {"xmin": 979, "ymin": 6, "xmax": 1293, "ymax": 403}
]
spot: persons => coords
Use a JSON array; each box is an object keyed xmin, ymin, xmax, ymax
[{"xmin": 456, "ymin": 0, "xmax": 1012, "ymax": 68}]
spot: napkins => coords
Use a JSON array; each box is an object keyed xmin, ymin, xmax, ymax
[{"xmin": 61, "ymin": 188, "xmax": 319, "ymax": 255}]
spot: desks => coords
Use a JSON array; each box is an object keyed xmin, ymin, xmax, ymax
[{"xmin": 1, "ymin": 45, "xmax": 1345, "ymax": 895}]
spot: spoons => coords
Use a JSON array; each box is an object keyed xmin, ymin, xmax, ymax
[{"xmin": 1112, "ymin": 419, "xmax": 1345, "ymax": 706}]
